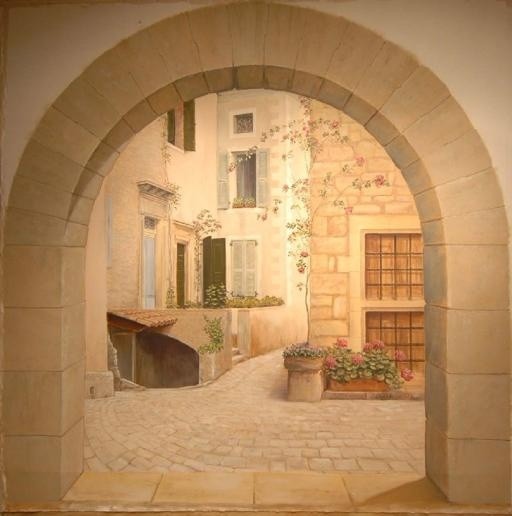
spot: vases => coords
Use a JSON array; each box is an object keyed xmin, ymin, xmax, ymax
[
  {"xmin": 284, "ymin": 355, "xmax": 326, "ymax": 402},
  {"xmin": 330, "ymin": 379, "xmax": 392, "ymax": 392}
]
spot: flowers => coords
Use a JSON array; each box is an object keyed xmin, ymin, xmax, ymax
[
  {"xmin": 326, "ymin": 337, "xmax": 414, "ymax": 388},
  {"xmin": 282, "ymin": 342, "xmax": 329, "ymax": 357}
]
